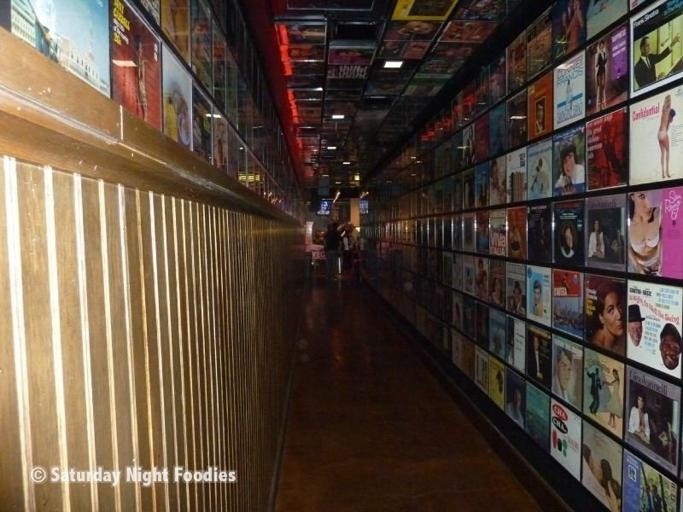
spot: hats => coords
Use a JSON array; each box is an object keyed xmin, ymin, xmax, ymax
[{"xmin": 627, "ymin": 303, "xmax": 647, "ymax": 322}]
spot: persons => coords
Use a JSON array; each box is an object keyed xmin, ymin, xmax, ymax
[
  {"xmin": 133, "ymin": 33, "xmax": 150, "ymax": 123},
  {"xmin": 383, "ymin": 20, "xmax": 442, "ymax": 42},
  {"xmin": 453, "ymin": 0, "xmax": 682, "ymax": 512},
  {"xmin": 314, "ymin": 221, "xmax": 359, "ymax": 283}
]
